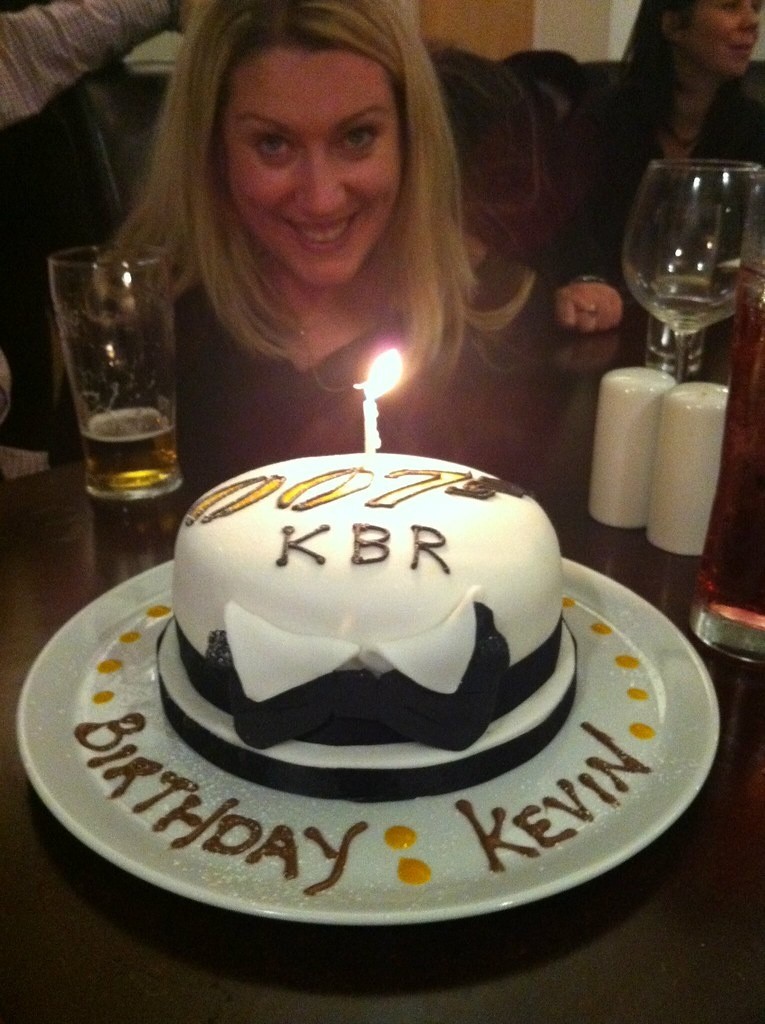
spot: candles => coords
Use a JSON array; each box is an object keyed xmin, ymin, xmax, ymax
[{"xmin": 352, "ymin": 348, "xmax": 411, "ymax": 471}]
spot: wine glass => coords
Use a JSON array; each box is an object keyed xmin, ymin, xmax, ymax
[{"xmin": 619, "ymin": 158, "xmax": 764, "ymax": 387}]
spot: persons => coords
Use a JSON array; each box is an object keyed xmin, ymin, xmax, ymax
[{"xmin": 0, "ymin": 0, "xmax": 765, "ymax": 490}]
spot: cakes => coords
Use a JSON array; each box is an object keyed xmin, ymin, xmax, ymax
[{"xmin": 156, "ymin": 453, "xmax": 578, "ymax": 803}]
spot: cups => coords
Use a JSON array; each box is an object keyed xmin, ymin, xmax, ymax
[
  {"xmin": 47, "ymin": 244, "xmax": 185, "ymax": 501},
  {"xmin": 685, "ymin": 180, "xmax": 764, "ymax": 672},
  {"xmin": 642, "ymin": 195, "xmax": 725, "ymax": 377}
]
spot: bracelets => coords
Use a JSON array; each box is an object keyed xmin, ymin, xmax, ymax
[{"xmin": 574, "ymin": 274, "xmax": 605, "ymax": 283}]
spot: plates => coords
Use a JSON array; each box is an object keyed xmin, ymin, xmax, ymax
[{"xmin": 13, "ymin": 555, "xmax": 722, "ymax": 928}]
